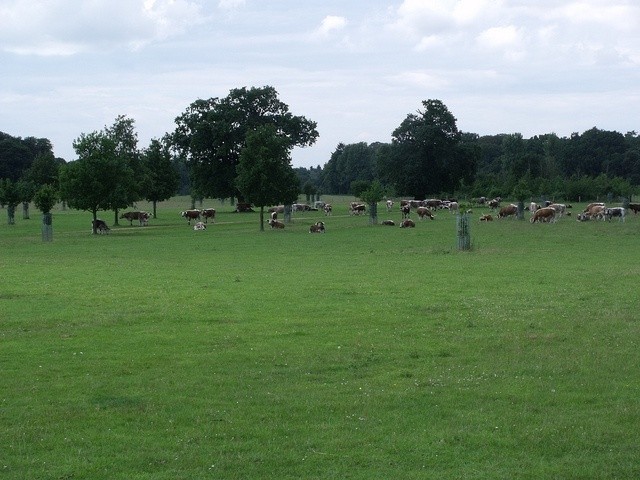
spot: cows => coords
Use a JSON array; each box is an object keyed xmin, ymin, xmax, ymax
[
  {"xmin": 232, "ymin": 209, "xmax": 239, "ymax": 213},
  {"xmin": 400, "ymin": 200, "xmax": 407, "ymax": 207},
  {"xmin": 314, "ymin": 201, "xmax": 325, "ymax": 210},
  {"xmin": 381, "ymin": 219, "xmax": 395, "ymax": 226},
  {"xmin": 201, "ymin": 208, "xmax": 216, "ymax": 223},
  {"xmin": 581, "ymin": 203, "xmax": 605, "ymax": 212},
  {"xmin": 480, "ymin": 214, "xmax": 493, "ymax": 221},
  {"xmin": 408, "ymin": 200, "xmax": 427, "ymax": 210},
  {"xmin": 547, "ymin": 204, "xmax": 566, "ymax": 219},
  {"xmin": 576, "ymin": 213, "xmax": 585, "ymax": 222},
  {"xmin": 496, "ymin": 204, "xmax": 518, "ymax": 220},
  {"xmin": 471, "ymin": 196, "xmax": 486, "ymax": 205},
  {"xmin": 193, "ymin": 222, "xmax": 207, "ymax": 231},
  {"xmin": 119, "ymin": 212, "xmax": 140, "ymax": 227},
  {"xmin": 425, "ymin": 199, "xmax": 444, "ymax": 211},
  {"xmin": 486, "ymin": 200, "xmax": 499, "ymax": 211},
  {"xmin": 350, "ymin": 202, "xmax": 359, "ymax": 213},
  {"xmin": 565, "ymin": 212, "xmax": 572, "ymax": 216},
  {"xmin": 629, "ymin": 204, "xmax": 640, "ymax": 215},
  {"xmin": 583, "ymin": 206, "xmax": 607, "ymax": 222},
  {"xmin": 567, "ymin": 203, "xmax": 572, "ymax": 208},
  {"xmin": 292, "ymin": 203, "xmax": 312, "ymax": 216},
  {"xmin": 417, "ymin": 206, "xmax": 436, "ymax": 220},
  {"xmin": 353, "ymin": 204, "xmax": 366, "ymax": 215},
  {"xmin": 529, "ymin": 202, "xmax": 541, "ymax": 212},
  {"xmin": 530, "ymin": 208, "xmax": 556, "ymax": 224},
  {"xmin": 401, "ymin": 205, "xmax": 411, "ymax": 219},
  {"xmin": 92, "ymin": 219, "xmax": 111, "ymax": 235},
  {"xmin": 524, "ymin": 206, "xmax": 529, "ymax": 210},
  {"xmin": 544, "ymin": 200, "xmax": 551, "ymax": 207},
  {"xmin": 400, "ymin": 219, "xmax": 416, "ymax": 228},
  {"xmin": 178, "ymin": 210, "xmax": 201, "ymax": 226},
  {"xmin": 138, "ymin": 211, "xmax": 154, "ymax": 227},
  {"xmin": 309, "ymin": 221, "xmax": 326, "ymax": 234},
  {"xmin": 325, "ymin": 204, "xmax": 333, "ymax": 216},
  {"xmin": 386, "ymin": 200, "xmax": 395, "ymax": 209},
  {"xmin": 605, "ymin": 207, "xmax": 626, "ymax": 223},
  {"xmin": 465, "ymin": 209, "xmax": 473, "ymax": 214},
  {"xmin": 446, "ymin": 202, "xmax": 460, "ymax": 215},
  {"xmin": 266, "ymin": 218, "xmax": 285, "ymax": 229},
  {"xmin": 271, "ymin": 212, "xmax": 277, "ymax": 219}
]
